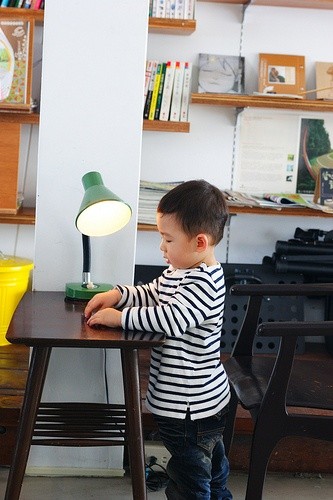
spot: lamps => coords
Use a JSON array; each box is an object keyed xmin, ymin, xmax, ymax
[{"xmin": 65, "ymin": 172, "xmax": 132, "ymax": 300}]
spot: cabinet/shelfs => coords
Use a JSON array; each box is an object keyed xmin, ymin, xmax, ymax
[{"xmin": 0, "ymin": 0, "xmax": 333, "ymax": 231}]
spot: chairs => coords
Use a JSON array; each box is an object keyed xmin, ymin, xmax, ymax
[{"xmin": 221, "ymin": 282, "xmax": 333, "ymax": 500}]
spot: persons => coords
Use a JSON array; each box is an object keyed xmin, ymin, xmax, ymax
[
  {"xmin": 270, "ymin": 68, "xmax": 284, "ymax": 82},
  {"xmin": 84, "ymin": 179, "xmax": 232, "ymax": 500}
]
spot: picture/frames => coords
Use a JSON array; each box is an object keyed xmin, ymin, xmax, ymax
[
  {"xmin": 258, "ymin": 53, "xmax": 306, "ymax": 98},
  {"xmin": 0, "ymin": 17, "xmax": 35, "ymax": 112}
]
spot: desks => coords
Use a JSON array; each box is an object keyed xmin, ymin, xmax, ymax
[{"xmin": 4, "ymin": 291, "xmax": 166, "ymax": 500}]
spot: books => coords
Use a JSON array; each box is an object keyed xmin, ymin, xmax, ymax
[
  {"xmin": 1, "ymin": 0, "xmax": 42, "ymax": 9},
  {"xmin": 144, "ymin": 60, "xmax": 191, "ymax": 122},
  {"xmin": 138, "ymin": 180, "xmax": 184, "ymax": 226},
  {"xmin": 149, "ymin": 0, "xmax": 193, "ymax": 19}
]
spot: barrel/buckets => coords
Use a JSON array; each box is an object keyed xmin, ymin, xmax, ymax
[{"xmin": 0, "ymin": 256, "xmax": 34, "ymax": 346}]
